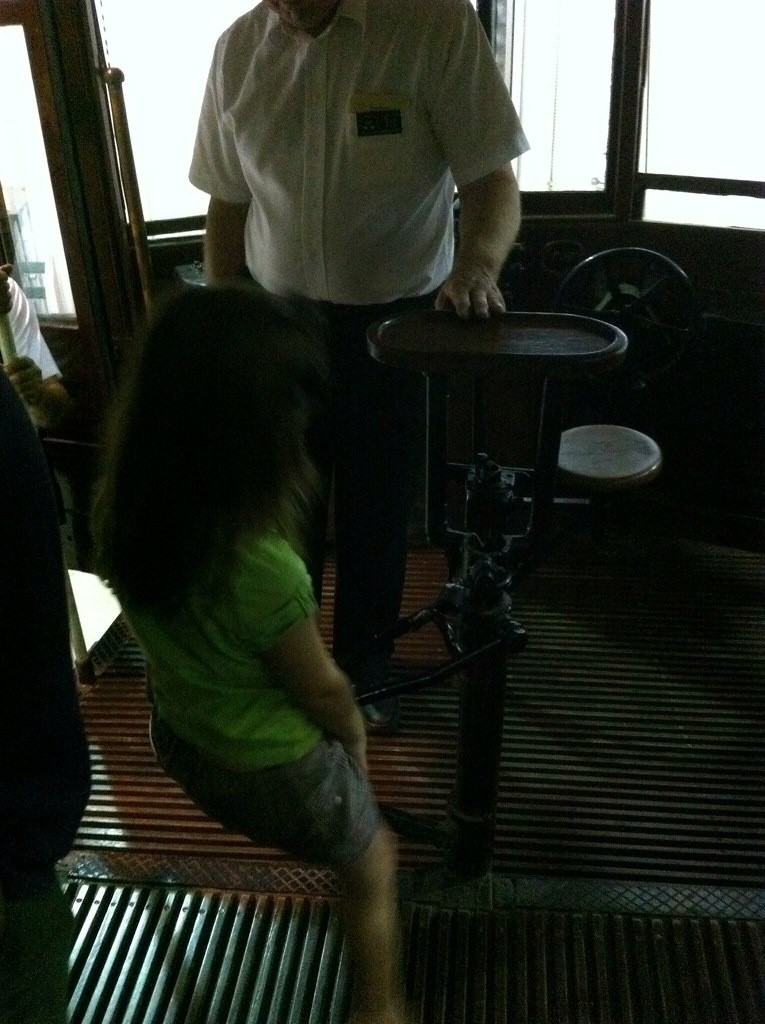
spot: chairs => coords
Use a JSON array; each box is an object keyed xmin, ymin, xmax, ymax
[{"xmin": 514, "ymin": 242, "xmax": 702, "ymax": 618}]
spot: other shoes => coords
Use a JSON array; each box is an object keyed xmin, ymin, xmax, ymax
[{"xmin": 357, "ymin": 694, "xmax": 400, "ymax": 735}]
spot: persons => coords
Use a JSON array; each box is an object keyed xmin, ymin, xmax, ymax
[
  {"xmin": 0, "ymin": 196, "xmax": 407, "ymax": 1024},
  {"xmin": 190, "ymin": 0, "xmax": 529, "ymax": 731}
]
